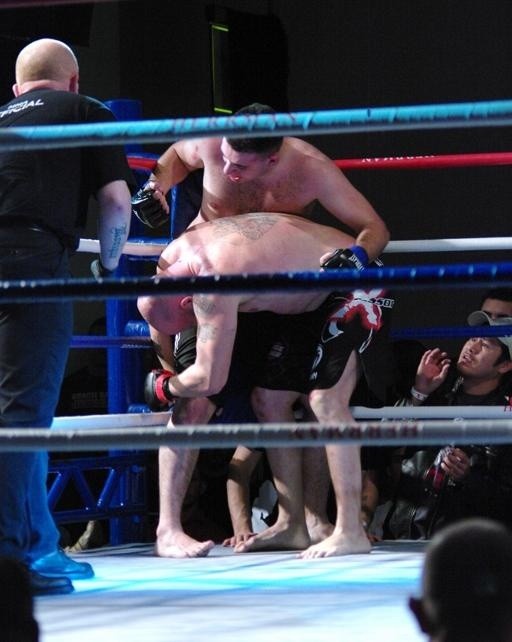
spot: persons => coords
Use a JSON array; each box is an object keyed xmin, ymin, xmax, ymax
[
  {"xmin": 130, "ymin": 102, "xmax": 390, "ymax": 558},
  {"xmin": 0, "ymin": 556, "xmax": 39, "ymax": 642},
  {"xmin": 136, "ymin": 212, "xmax": 387, "ymax": 559},
  {"xmin": 55, "ymin": 316, "xmax": 111, "ymax": 546},
  {"xmin": 407, "ymin": 522, "xmax": 511, "ymax": 642},
  {"xmin": 141, "ymin": 461, "xmax": 228, "ymax": 542},
  {"xmin": 0, "ymin": 36, "xmax": 134, "ymax": 596},
  {"xmin": 224, "ymin": 291, "xmax": 511, "ymax": 540}
]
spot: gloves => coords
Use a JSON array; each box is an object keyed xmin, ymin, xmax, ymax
[{"xmin": 143, "ymin": 368, "xmax": 179, "ymax": 413}]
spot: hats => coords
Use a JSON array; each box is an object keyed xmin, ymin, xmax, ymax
[{"xmin": 467, "ymin": 311, "xmax": 511, "ymax": 361}]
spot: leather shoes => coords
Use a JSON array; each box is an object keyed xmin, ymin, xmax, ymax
[
  {"xmin": 33, "ymin": 547, "xmax": 94, "ymax": 580},
  {"xmin": 26, "ymin": 568, "xmax": 74, "ymax": 595}
]
